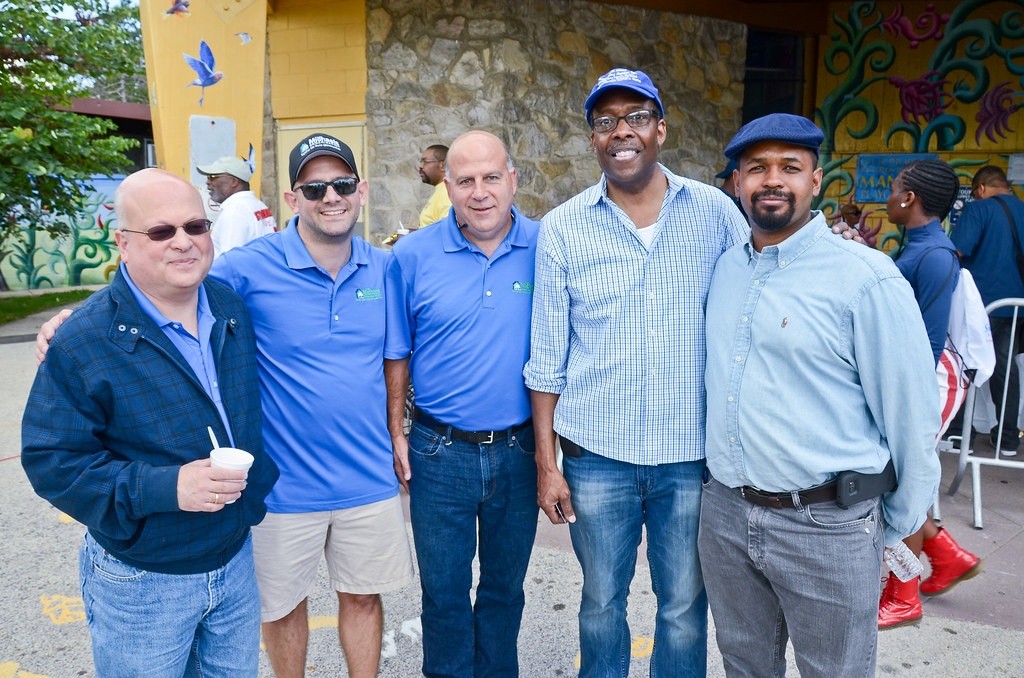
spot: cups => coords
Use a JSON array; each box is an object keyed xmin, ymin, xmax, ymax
[
  {"xmin": 397, "ymin": 229, "xmax": 409, "ymax": 235},
  {"xmin": 884, "ymin": 540, "xmax": 924, "ymax": 582},
  {"xmin": 210, "ymin": 448, "xmax": 254, "ymax": 504}
]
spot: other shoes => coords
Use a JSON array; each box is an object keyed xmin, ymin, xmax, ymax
[
  {"xmin": 940, "ymin": 432, "xmax": 974, "ymax": 455},
  {"xmin": 990, "ymin": 436, "xmax": 1017, "ymax": 456}
]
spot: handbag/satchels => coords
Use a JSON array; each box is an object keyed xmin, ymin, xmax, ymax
[
  {"xmin": 971, "ymin": 379, "xmax": 998, "ymax": 434},
  {"xmin": 1016, "ymin": 353, "xmax": 1024, "ymax": 431},
  {"xmin": 944, "ymin": 268, "xmax": 997, "ymax": 389}
]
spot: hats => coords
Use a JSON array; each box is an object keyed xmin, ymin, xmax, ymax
[
  {"xmin": 582, "ymin": 67, "xmax": 664, "ymax": 128},
  {"xmin": 288, "ymin": 133, "xmax": 360, "ymax": 191},
  {"xmin": 196, "ymin": 157, "xmax": 252, "ymax": 183},
  {"xmin": 724, "ymin": 112, "xmax": 825, "ymax": 151},
  {"xmin": 714, "ymin": 160, "xmax": 740, "ymax": 179}
]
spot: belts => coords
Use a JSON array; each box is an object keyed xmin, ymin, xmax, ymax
[
  {"xmin": 738, "ymin": 476, "xmax": 839, "ymax": 509},
  {"xmin": 410, "ymin": 406, "xmax": 534, "ymax": 445}
]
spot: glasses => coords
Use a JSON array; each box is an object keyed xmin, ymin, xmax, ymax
[
  {"xmin": 121, "ymin": 218, "xmax": 213, "ymax": 241},
  {"xmin": 293, "ymin": 177, "xmax": 358, "ymax": 202},
  {"xmin": 591, "ymin": 108, "xmax": 659, "ymax": 139},
  {"xmin": 205, "ymin": 174, "xmax": 241, "ymax": 184},
  {"xmin": 418, "ymin": 158, "xmax": 445, "ymax": 166},
  {"xmin": 969, "ymin": 183, "xmax": 985, "ymax": 198}
]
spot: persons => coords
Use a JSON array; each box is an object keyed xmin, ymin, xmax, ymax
[
  {"xmin": 878, "ymin": 159, "xmax": 983, "ymax": 631},
  {"xmin": 933, "ymin": 165, "xmax": 1024, "ymax": 457},
  {"xmin": 841, "ymin": 204, "xmax": 860, "ymax": 229},
  {"xmin": 383, "ymin": 130, "xmax": 541, "ymax": 677},
  {"xmin": 36, "ymin": 129, "xmax": 414, "ymax": 678},
  {"xmin": 22, "ymin": 167, "xmax": 279, "ymax": 678},
  {"xmin": 696, "ymin": 114, "xmax": 941, "ymax": 677},
  {"xmin": 523, "ymin": 67, "xmax": 870, "ymax": 677},
  {"xmin": 714, "ymin": 161, "xmax": 750, "ymax": 228},
  {"xmin": 416, "ymin": 145, "xmax": 453, "ymax": 230},
  {"xmin": 195, "ymin": 157, "xmax": 278, "ymax": 260}
]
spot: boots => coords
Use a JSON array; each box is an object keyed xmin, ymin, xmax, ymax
[
  {"xmin": 921, "ymin": 525, "xmax": 981, "ymax": 594},
  {"xmin": 877, "ymin": 571, "xmax": 923, "ymax": 631}
]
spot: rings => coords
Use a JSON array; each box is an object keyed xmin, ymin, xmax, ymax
[{"xmin": 214, "ymin": 493, "xmax": 218, "ymax": 505}]
